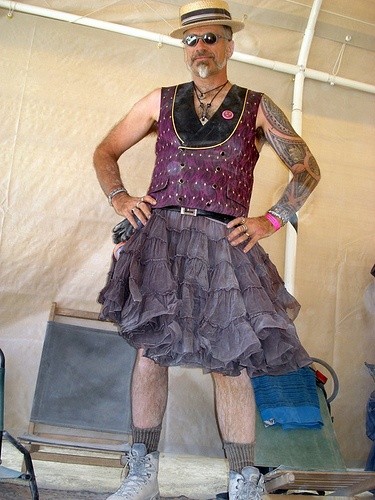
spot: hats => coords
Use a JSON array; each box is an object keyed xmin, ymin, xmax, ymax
[{"xmin": 169, "ymin": 0, "xmax": 245, "ymax": 39}]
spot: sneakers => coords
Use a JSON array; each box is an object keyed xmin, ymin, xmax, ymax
[
  {"xmin": 228, "ymin": 464, "xmax": 265, "ymax": 499},
  {"xmin": 106, "ymin": 442, "xmax": 160, "ymax": 500}
]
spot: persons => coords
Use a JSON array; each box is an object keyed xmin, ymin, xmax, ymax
[{"xmin": 92, "ymin": 0, "xmax": 320, "ymax": 500}]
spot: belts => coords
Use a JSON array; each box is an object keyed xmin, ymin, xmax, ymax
[{"xmin": 171, "ymin": 204, "xmax": 236, "ymax": 222}]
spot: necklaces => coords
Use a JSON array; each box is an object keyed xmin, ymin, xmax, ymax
[{"xmin": 192, "ymin": 80, "xmax": 228, "ymax": 126}]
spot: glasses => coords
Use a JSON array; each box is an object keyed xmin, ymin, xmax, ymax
[{"xmin": 182, "ymin": 33, "xmax": 232, "ymax": 46}]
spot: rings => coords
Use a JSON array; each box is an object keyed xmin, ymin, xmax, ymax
[
  {"xmin": 131, "ymin": 208, "xmax": 137, "ymax": 214},
  {"xmin": 246, "ymin": 233, "xmax": 249, "ymax": 236},
  {"xmin": 242, "ymin": 224, "xmax": 247, "ymax": 231},
  {"xmin": 241, "ymin": 217, "xmax": 245, "ymax": 224},
  {"xmin": 141, "ymin": 196, "xmax": 145, "ymax": 201},
  {"xmin": 137, "ymin": 201, "xmax": 142, "ymax": 208}
]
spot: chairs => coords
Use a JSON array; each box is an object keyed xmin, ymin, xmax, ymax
[
  {"xmin": 16, "ymin": 301, "xmax": 137, "ymax": 480},
  {"xmin": 254, "ymin": 355, "xmax": 375, "ymax": 500}
]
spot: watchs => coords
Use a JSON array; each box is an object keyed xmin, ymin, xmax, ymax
[{"xmin": 268, "ymin": 205, "xmax": 289, "ymax": 226}]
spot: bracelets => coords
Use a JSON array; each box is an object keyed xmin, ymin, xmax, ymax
[
  {"xmin": 108, "ymin": 188, "xmax": 127, "ymax": 206},
  {"xmin": 265, "ymin": 214, "xmax": 280, "ymax": 231}
]
